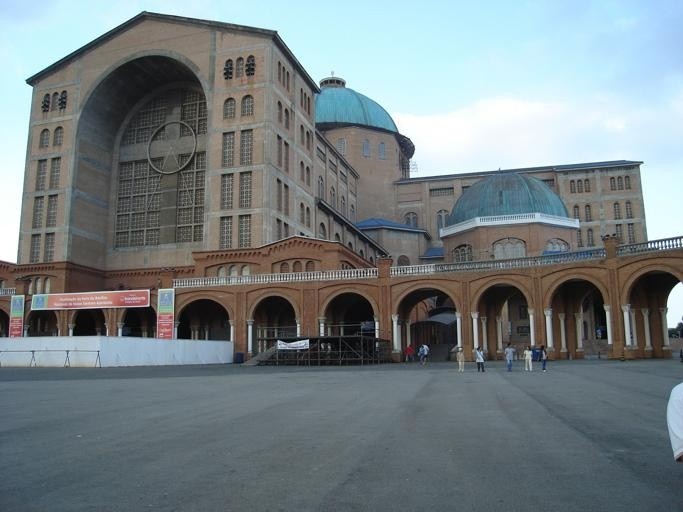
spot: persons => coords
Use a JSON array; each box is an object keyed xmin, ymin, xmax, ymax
[
  {"xmin": 475, "ymin": 346, "xmax": 485, "ymax": 372},
  {"xmin": 405, "ymin": 342, "xmax": 429, "ymax": 365},
  {"xmin": 666, "ymin": 382, "xmax": 683, "ymax": 461},
  {"xmin": 504, "ymin": 342, "xmax": 548, "ymax": 373},
  {"xmin": 456, "ymin": 347, "xmax": 465, "ymax": 373}
]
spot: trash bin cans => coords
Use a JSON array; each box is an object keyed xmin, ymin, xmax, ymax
[
  {"xmin": 235, "ymin": 352, "xmax": 243, "ymax": 363},
  {"xmin": 532, "ymin": 348, "xmax": 541, "ymax": 361}
]
